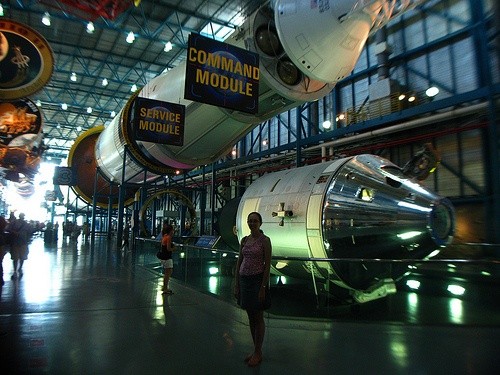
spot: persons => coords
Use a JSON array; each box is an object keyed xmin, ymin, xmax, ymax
[
  {"xmin": 235, "ymin": 212, "xmax": 272, "ymax": 367},
  {"xmin": 0, "ymin": 216, "xmax": 12, "ymax": 289},
  {"xmin": 12, "ymin": 213, "xmax": 32, "ymax": 272},
  {"xmin": 161, "ymin": 223, "xmax": 177, "ymax": 294},
  {"xmin": 0, "ymin": 211, "xmax": 204, "ymax": 253}
]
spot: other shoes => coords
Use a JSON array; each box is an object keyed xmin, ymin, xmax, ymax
[
  {"xmin": 244, "ymin": 352, "xmax": 255, "ymax": 363},
  {"xmin": 248, "ymin": 356, "xmax": 263, "ymax": 366}
]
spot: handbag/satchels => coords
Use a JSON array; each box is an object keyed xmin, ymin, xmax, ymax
[
  {"xmin": 256, "ymin": 288, "xmax": 273, "ymax": 310},
  {"xmin": 156, "ymin": 251, "xmax": 168, "ymax": 260}
]
